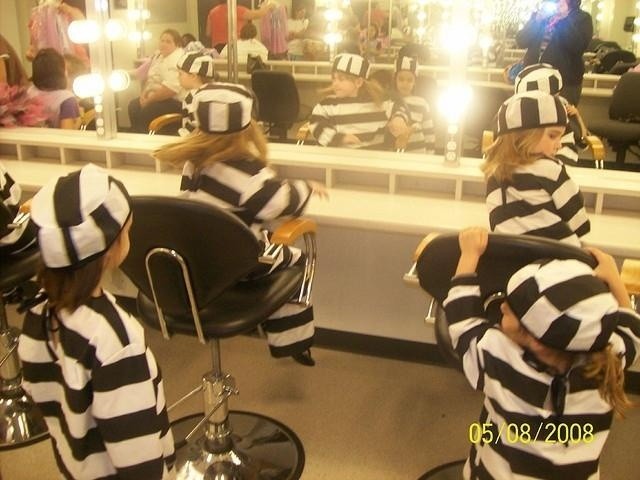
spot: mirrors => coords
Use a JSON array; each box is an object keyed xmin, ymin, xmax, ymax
[
  {"xmin": 0, "ymin": 1, "xmax": 639, "ymax": 171},
  {"xmin": 138, "ymin": 1, "xmax": 639, "ymax": 74}
]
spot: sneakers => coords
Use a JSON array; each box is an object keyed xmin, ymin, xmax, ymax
[{"xmin": 293, "ymin": 349, "xmax": 314, "ymax": 366}]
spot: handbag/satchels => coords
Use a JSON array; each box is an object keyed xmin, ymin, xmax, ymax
[{"xmin": 503, "ymin": 61, "xmax": 529, "ymax": 84}]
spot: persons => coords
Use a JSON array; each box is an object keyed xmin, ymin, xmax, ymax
[
  {"xmin": 25, "ymin": 48, "xmax": 82, "ymax": 131},
  {"xmin": 435, "ymin": 223, "xmax": 639, "ymax": 480},
  {"xmin": 150, "ymin": 81, "xmax": 318, "ymax": 360},
  {"xmin": 517, "ymin": 62, "xmax": 589, "ymax": 162},
  {"xmin": 478, "ymin": 90, "xmax": 593, "ymax": 256},
  {"xmin": 1, "ymin": 164, "xmax": 42, "ymax": 263},
  {"xmin": 514, "ymin": 0, "xmax": 594, "ymax": 107},
  {"xmin": 16, "ymin": 163, "xmax": 181, "ymax": 479},
  {"xmin": 127, "ymin": 2, "xmax": 453, "ymax": 153}
]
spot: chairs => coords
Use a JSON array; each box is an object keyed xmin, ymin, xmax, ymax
[{"xmin": 405, "ymin": 233, "xmax": 640, "ymax": 479}]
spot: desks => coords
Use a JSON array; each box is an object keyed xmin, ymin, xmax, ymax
[{"xmin": 0, "ymin": 123, "xmax": 640, "ymax": 393}]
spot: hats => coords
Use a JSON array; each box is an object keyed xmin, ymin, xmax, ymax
[
  {"xmin": 507, "ymin": 257, "xmax": 619, "ymax": 351},
  {"xmin": 515, "ymin": 63, "xmax": 563, "ymax": 94},
  {"xmin": 177, "ymin": 52, "xmax": 214, "ymax": 78},
  {"xmin": 331, "ymin": 53, "xmax": 370, "ymax": 79},
  {"xmin": 32, "ymin": 162, "xmax": 133, "ymax": 269},
  {"xmin": 394, "ymin": 56, "xmax": 418, "ymax": 77},
  {"xmin": 192, "ymin": 82, "xmax": 254, "ymax": 135},
  {"xmin": 494, "ymin": 90, "xmax": 569, "ymax": 141}
]
